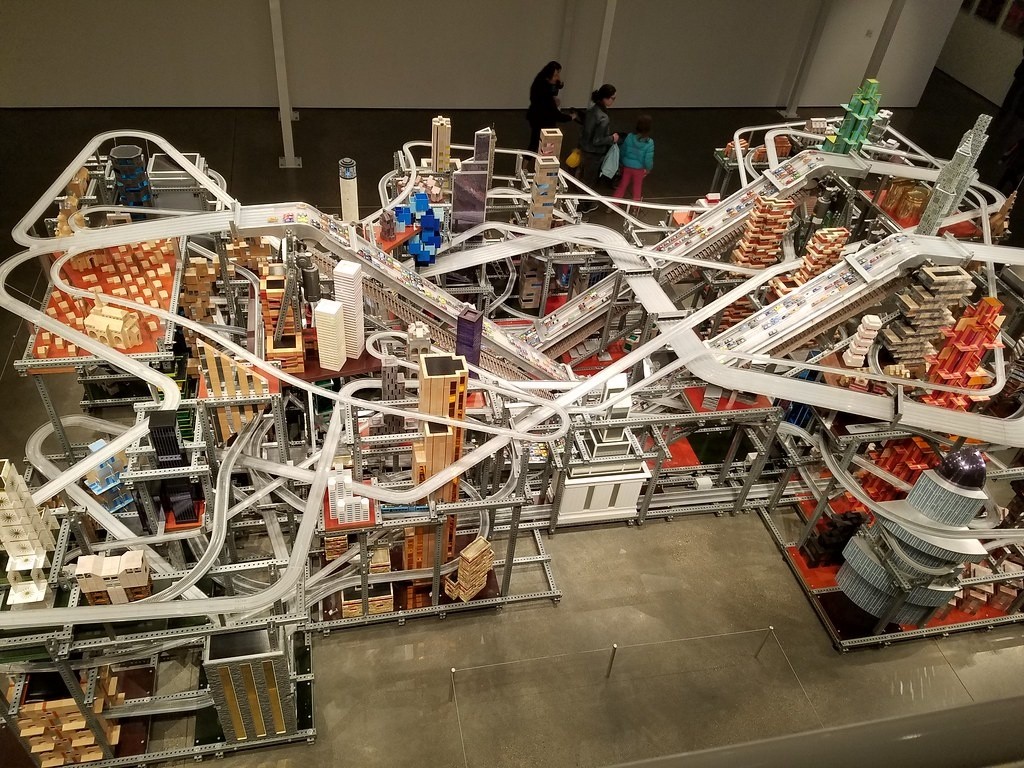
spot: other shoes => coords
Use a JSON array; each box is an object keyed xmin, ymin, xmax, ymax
[
  {"xmin": 605, "ymin": 207, "xmax": 613, "ymax": 214},
  {"xmin": 631, "ymin": 209, "xmax": 641, "ymax": 215}
]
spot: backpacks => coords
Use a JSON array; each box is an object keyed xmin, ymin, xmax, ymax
[{"xmin": 600, "ymin": 141, "xmax": 620, "ymax": 179}]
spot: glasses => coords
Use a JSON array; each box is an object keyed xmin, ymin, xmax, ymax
[{"xmin": 610, "ymin": 96, "xmax": 615, "ymax": 100}]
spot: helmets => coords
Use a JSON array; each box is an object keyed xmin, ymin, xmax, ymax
[{"xmin": 565, "ymin": 148, "xmax": 581, "ymax": 168}]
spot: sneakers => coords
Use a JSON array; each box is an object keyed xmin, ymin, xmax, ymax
[{"xmin": 581, "ymin": 204, "xmax": 598, "ymax": 213}]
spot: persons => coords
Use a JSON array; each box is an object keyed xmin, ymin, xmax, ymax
[
  {"xmin": 546, "ymin": 62, "xmax": 565, "ymax": 100},
  {"xmin": 521, "ymin": 61, "xmax": 575, "ymax": 172},
  {"xmin": 606, "ymin": 113, "xmax": 657, "ymax": 217},
  {"xmin": 575, "ymin": 83, "xmax": 620, "ymax": 214}
]
